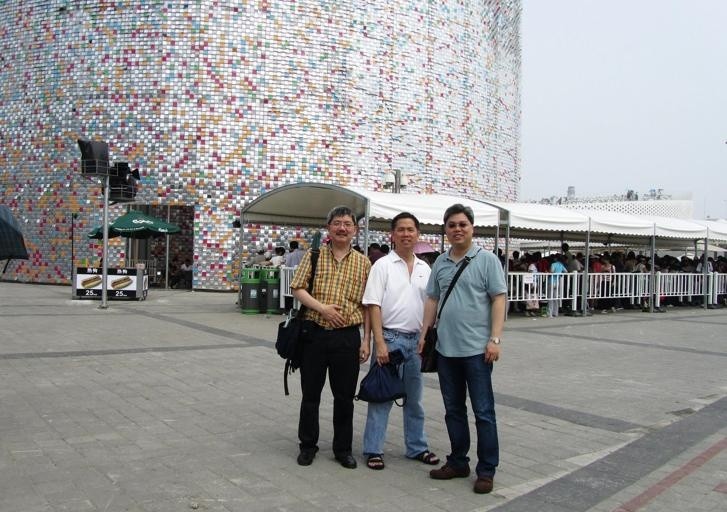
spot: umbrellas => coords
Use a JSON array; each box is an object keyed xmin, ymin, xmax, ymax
[{"xmin": 88, "ymin": 208, "xmax": 181, "ymax": 269}]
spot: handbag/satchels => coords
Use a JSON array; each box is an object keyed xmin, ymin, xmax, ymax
[
  {"xmin": 420, "ymin": 326, "xmax": 441, "ymax": 372},
  {"xmin": 354, "ymin": 349, "xmax": 407, "ymax": 407},
  {"xmin": 276, "ymin": 318, "xmax": 303, "ymax": 360}
]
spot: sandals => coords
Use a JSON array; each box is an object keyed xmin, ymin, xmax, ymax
[
  {"xmin": 367, "ymin": 455, "xmax": 384, "ymax": 469},
  {"xmin": 417, "ymin": 451, "xmax": 439, "ymax": 464}
]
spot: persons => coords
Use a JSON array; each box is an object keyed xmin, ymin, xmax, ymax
[
  {"xmin": 588, "ymin": 251, "xmax": 652, "ymax": 314},
  {"xmin": 655, "ymin": 253, "xmax": 727, "ymax": 308},
  {"xmin": 509, "ymin": 243, "xmax": 584, "ymax": 316},
  {"xmin": 290, "ymin": 205, "xmax": 373, "ymax": 469},
  {"xmin": 418, "ymin": 203, "xmax": 508, "ymax": 493},
  {"xmin": 360, "ymin": 212, "xmax": 439, "ymax": 469},
  {"xmin": 245, "ymin": 240, "xmax": 504, "ymax": 316}
]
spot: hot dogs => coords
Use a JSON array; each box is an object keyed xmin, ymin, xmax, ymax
[
  {"xmin": 110, "ymin": 275, "xmax": 133, "ymax": 291},
  {"xmin": 80, "ymin": 275, "xmax": 103, "ymax": 289}
]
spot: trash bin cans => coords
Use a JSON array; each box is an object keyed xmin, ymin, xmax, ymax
[
  {"xmin": 240, "ymin": 268, "xmax": 262, "ymax": 315},
  {"xmin": 263, "ymin": 267, "xmax": 280, "ymax": 315}
]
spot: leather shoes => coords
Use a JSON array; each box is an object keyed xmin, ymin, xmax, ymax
[
  {"xmin": 297, "ymin": 451, "xmax": 314, "ymax": 465},
  {"xmin": 336, "ymin": 455, "xmax": 356, "ymax": 469},
  {"xmin": 430, "ymin": 463, "xmax": 470, "ymax": 479},
  {"xmin": 474, "ymin": 476, "xmax": 493, "ymax": 493}
]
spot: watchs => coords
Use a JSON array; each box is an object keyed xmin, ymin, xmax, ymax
[{"xmin": 489, "ymin": 335, "xmax": 500, "ymax": 344}]
